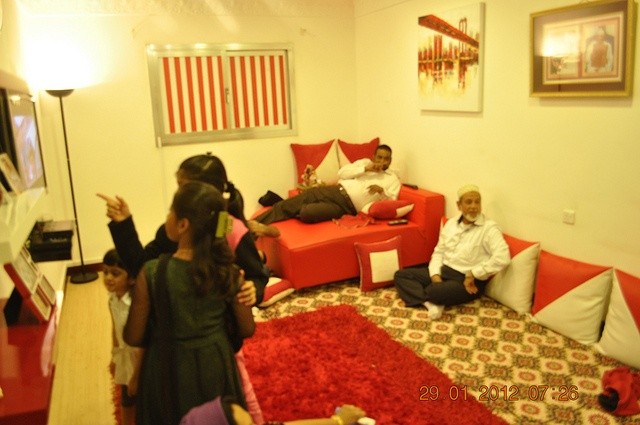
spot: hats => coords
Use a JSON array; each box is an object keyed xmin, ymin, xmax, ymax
[{"xmin": 457, "ymin": 184, "xmax": 479, "ymax": 200}]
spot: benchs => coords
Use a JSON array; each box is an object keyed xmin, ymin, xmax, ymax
[{"xmin": 250, "ymin": 184, "xmax": 445, "ymax": 289}]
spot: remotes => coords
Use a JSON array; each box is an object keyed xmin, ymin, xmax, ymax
[{"xmin": 388, "ymin": 220, "xmax": 408, "ymax": 225}]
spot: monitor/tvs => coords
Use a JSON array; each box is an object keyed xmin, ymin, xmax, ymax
[{"xmin": 0, "ymin": 87, "xmax": 46, "ymax": 190}]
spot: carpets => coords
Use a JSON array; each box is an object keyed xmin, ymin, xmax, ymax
[{"xmin": 242, "ymin": 303, "xmax": 509, "ymax": 425}]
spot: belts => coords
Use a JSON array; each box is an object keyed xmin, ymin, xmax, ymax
[{"xmin": 338, "ymin": 184, "xmax": 356, "ymax": 213}]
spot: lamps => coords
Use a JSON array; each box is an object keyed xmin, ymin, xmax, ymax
[{"xmin": 19, "ymin": 11, "xmax": 105, "ymax": 283}]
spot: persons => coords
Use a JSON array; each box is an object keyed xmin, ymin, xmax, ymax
[
  {"xmin": 252, "ymin": 145, "xmax": 400, "ymax": 224},
  {"xmin": 94, "ymin": 153, "xmax": 269, "ymax": 425},
  {"xmin": 394, "ymin": 182, "xmax": 511, "ymax": 321}
]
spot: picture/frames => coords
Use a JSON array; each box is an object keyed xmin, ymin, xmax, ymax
[
  {"xmin": 419, "ymin": 2, "xmax": 484, "ymax": 110},
  {"xmin": 530, "ymin": 0, "xmax": 638, "ymax": 97}
]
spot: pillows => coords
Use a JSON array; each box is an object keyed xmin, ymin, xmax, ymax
[
  {"xmin": 531, "ymin": 251, "xmax": 613, "ymax": 344},
  {"xmin": 256, "ymin": 277, "xmax": 294, "ymax": 308},
  {"xmin": 484, "ymin": 232, "xmax": 540, "ymax": 315},
  {"xmin": 591, "ymin": 269, "xmax": 640, "ymax": 370},
  {"xmin": 337, "ymin": 137, "xmax": 380, "ymax": 169},
  {"xmin": 291, "ymin": 138, "xmax": 340, "ymax": 188},
  {"xmin": 355, "ymin": 235, "xmax": 402, "ymax": 291},
  {"xmin": 360, "ymin": 199, "xmax": 415, "ymax": 218}
]
